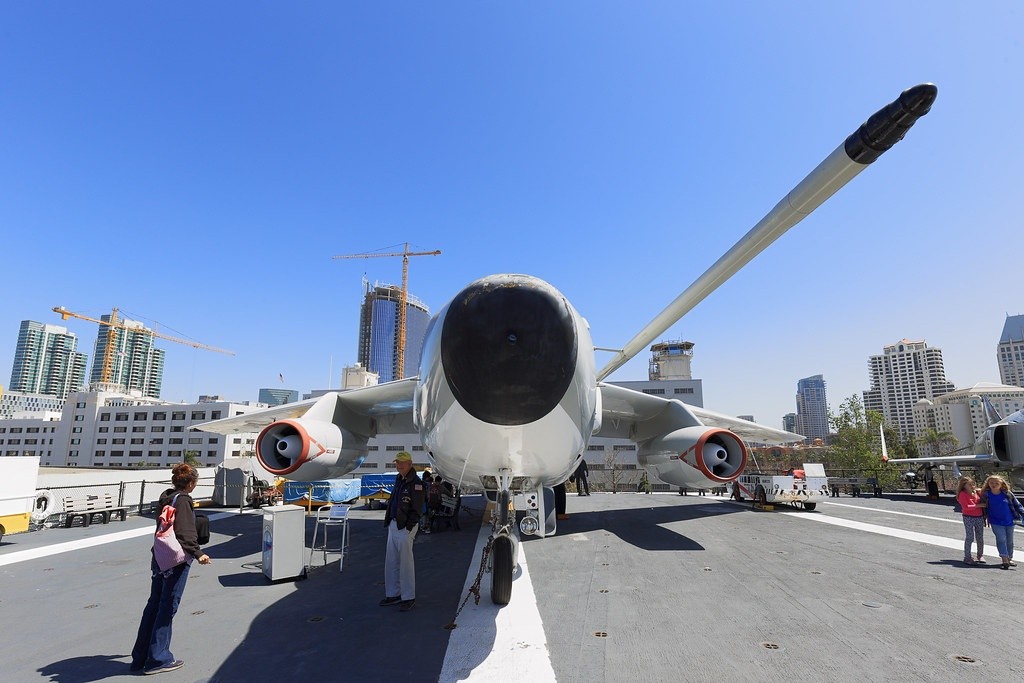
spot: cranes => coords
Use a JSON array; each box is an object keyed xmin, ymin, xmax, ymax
[
  {"xmin": 52, "ymin": 306, "xmax": 233, "ymax": 382},
  {"xmin": 332, "ymin": 243, "xmax": 441, "ymax": 381}
]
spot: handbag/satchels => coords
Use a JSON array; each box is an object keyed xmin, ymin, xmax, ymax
[
  {"xmin": 153, "ymin": 493, "xmax": 191, "ymax": 573},
  {"xmin": 569, "ymin": 475, "xmax": 575, "ymax": 483},
  {"xmin": 1004, "ymin": 491, "xmax": 1022, "ymax": 520}
]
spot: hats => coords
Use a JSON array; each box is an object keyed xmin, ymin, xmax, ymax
[{"xmin": 393, "ymin": 451, "xmax": 411, "ymax": 462}]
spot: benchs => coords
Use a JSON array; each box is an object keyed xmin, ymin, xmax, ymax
[{"xmin": 62, "ymin": 493, "xmax": 129, "ymax": 527}]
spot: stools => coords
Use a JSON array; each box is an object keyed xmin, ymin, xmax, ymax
[{"xmin": 308, "ymin": 503, "xmax": 352, "ymax": 573}]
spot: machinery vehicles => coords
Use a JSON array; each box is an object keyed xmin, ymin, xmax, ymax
[
  {"xmin": 246, "ymin": 473, "xmax": 280, "ymax": 509},
  {"xmin": 733, "ymin": 459, "xmax": 828, "ymax": 511}
]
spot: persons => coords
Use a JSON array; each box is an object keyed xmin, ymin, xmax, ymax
[
  {"xmin": 129, "ymin": 463, "xmax": 211, "ymax": 674},
  {"xmin": 574, "ymin": 459, "xmax": 590, "ymax": 496},
  {"xmin": 979, "ymin": 474, "xmax": 1024, "ymax": 568},
  {"xmin": 417, "ymin": 471, "xmax": 452, "ymax": 533},
  {"xmin": 553, "ymin": 482, "xmax": 568, "ymax": 520},
  {"xmin": 379, "ymin": 451, "xmax": 425, "ymax": 611},
  {"xmin": 957, "ymin": 476, "xmax": 987, "ymax": 565}
]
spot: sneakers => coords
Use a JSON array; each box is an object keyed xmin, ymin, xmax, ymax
[
  {"xmin": 379, "ymin": 594, "xmax": 401, "ymax": 606},
  {"xmin": 130, "ymin": 660, "xmax": 143, "ymax": 671},
  {"xmin": 143, "ymin": 658, "xmax": 185, "ymax": 674},
  {"xmin": 399, "ymin": 599, "xmax": 415, "ymax": 611}
]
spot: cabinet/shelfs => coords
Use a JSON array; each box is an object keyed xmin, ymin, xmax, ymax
[{"xmin": 262, "ymin": 503, "xmax": 305, "ymax": 581}]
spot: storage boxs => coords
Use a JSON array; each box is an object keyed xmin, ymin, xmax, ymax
[{"xmin": 763, "ymin": 505, "xmax": 774, "ymax": 510}]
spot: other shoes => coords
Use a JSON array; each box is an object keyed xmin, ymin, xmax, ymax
[
  {"xmin": 1009, "ymin": 558, "xmax": 1018, "ymax": 566},
  {"xmin": 964, "ymin": 557, "xmax": 977, "ymax": 566},
  {"xmin": 1002, "ymin": 557, "xmax": 1010, "ymax": 569},
  {"xmin": 558, "ymin": 514, "xmax": 569, "ymax": 520},
  {"xmin": 978, "ymin": 557, "xmax": 986, "ymax": 563}
]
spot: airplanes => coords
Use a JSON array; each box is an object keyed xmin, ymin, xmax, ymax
[
  {"xmin": 187, "ymin": 81, "xmax": 938, "ymax": 605},
  {"xmin": 879, "ymin": 393, "xmax": 1024, "ymax": 500}
]
